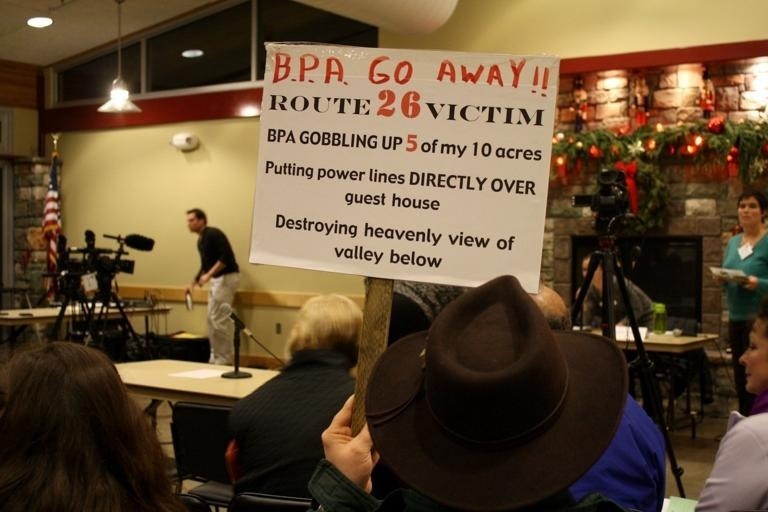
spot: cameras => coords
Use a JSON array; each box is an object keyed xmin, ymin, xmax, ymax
[{"xmin": 570, "ymin": 167, "xmax": 630, "ymax": 230}]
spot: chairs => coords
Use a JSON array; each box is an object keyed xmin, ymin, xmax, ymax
[{"xmin": 169, "ymin": 402, "xmax": 312, "ymax": 512}]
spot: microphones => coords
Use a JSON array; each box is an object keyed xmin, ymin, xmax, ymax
[{"xmin": 219, "ymin": 302, "xmax": 253, "ymax": 336}]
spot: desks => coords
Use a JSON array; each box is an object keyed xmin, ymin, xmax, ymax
[
  {"xmin": 592, "ymin": 329, "xmax": 719, "ymax": 440},
  {"xmin": 115, "ymin": 359, "xmax": 279, "ymax": 426},
  {"xmin": 0, "ymin": 303, "xmax": 170, "ymax": 341}
]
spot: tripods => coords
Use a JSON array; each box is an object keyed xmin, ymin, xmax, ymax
[
  {"xmin": 570, "ymin": 247, "xmax": 686, "ymax": 498},
  {"xmin": 48, "ymin": 294, "xmax": 147, "ymax": 360}
]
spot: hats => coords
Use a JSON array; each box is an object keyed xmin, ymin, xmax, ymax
[{"xmin": 364, "ymin": 275, "xmax": 629, "ymax": 512}]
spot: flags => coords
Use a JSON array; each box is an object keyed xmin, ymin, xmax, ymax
[{"xmin": 42, "ymin": 156, "xmax": 62, "ymax": 305}]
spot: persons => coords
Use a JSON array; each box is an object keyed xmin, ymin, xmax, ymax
[
  {"xmin": 185, "ymin": 209, "xmax": 241, "ymax": 366},
  {"xmin": 307, "ymin": 274, "xmax": 630, "ymax": 512},
  {"xmin": 225, "ymin": 294, "xmax": 364, "ymax": 512},
  {"xmin": 694, "ymin": 296, "xmax": 768, "ymax": 512},
  {"xmin": 0, "ymin": 340, "xmax": 188, "ymax": 512},
  {"xmin": 573, "ymin": 254, "xmax": 664, "ymax": 424},
  {"xmin": 529, "ymin": 285, "xmax": 667, "ymax": 512},
  {"xmin": 712, "ymin": 188, "xmax": 768, "ymax": 416}
]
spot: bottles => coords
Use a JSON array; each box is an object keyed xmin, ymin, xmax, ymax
[
  {"xmin": 652, "ymin": 303, "xmax": 668, "ymax": 334},
  {"xmin": 184, "ymin": 289, "xmax": 193, "ymax": 311}
]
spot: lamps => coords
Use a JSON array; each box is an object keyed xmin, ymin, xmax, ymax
[{"xmin": 97, "ymin": 0, "xmax": 142, "ymax": 114}]
